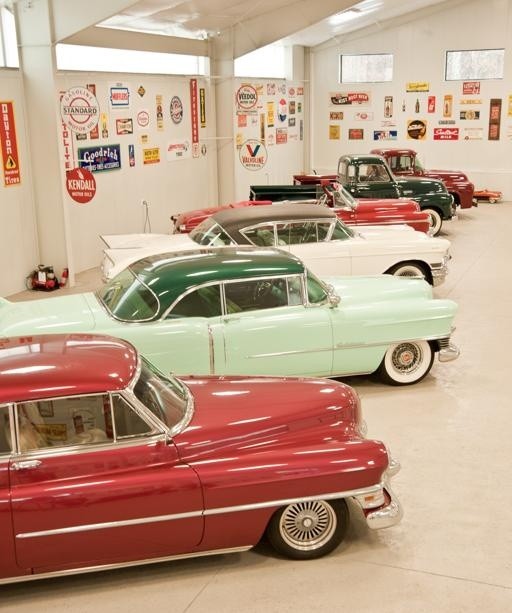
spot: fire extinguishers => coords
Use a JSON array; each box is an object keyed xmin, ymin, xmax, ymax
[{"xmin": 59, "ymin": 267, "xmax": 68, "ymax": 287}]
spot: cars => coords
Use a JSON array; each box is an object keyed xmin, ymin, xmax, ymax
[
  {"xmin": 0, "ymin": 330, "xmax": 402, "ymax": 593},
  {"xmin": 94, "ymin": 202, "xmax": 453, "ymax": 297},
  {"xmin": 295, "ymin": 148, "xmax": 479, "ymax": 209},
  {"xmin": 0, "ymin": 245, "xmax": 462, "ymax": 385}
]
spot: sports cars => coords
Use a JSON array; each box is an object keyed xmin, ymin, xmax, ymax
[{"xmin": 169, "ymin": 182, "xmax": 433, "ymax": 236}]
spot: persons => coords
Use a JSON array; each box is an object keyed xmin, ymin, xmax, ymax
[{"xmin": 365, "ymin": 165, "xmax": 385, "ymax": 181}]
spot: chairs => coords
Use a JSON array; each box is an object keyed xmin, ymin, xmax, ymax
[
  {"xmin": 21, "ymin": 400, "xmax": 75, "ymax": 445},
  {"xmin": 1, "ymin": 405, "xmax": 107, "ymax": 451},
  {"xmin": 196, "ymin": 284, "xmax": 259, "ymax": 314}
]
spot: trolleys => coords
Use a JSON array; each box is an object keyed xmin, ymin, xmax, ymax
[{"xmin": 471, "ymin": 188, "xmax": 506, "ymax": 203}]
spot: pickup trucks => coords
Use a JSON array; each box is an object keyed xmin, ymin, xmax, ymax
[{"xmin": 247, "ymin": 153, "xmax": 456, "ymax": 235}]
